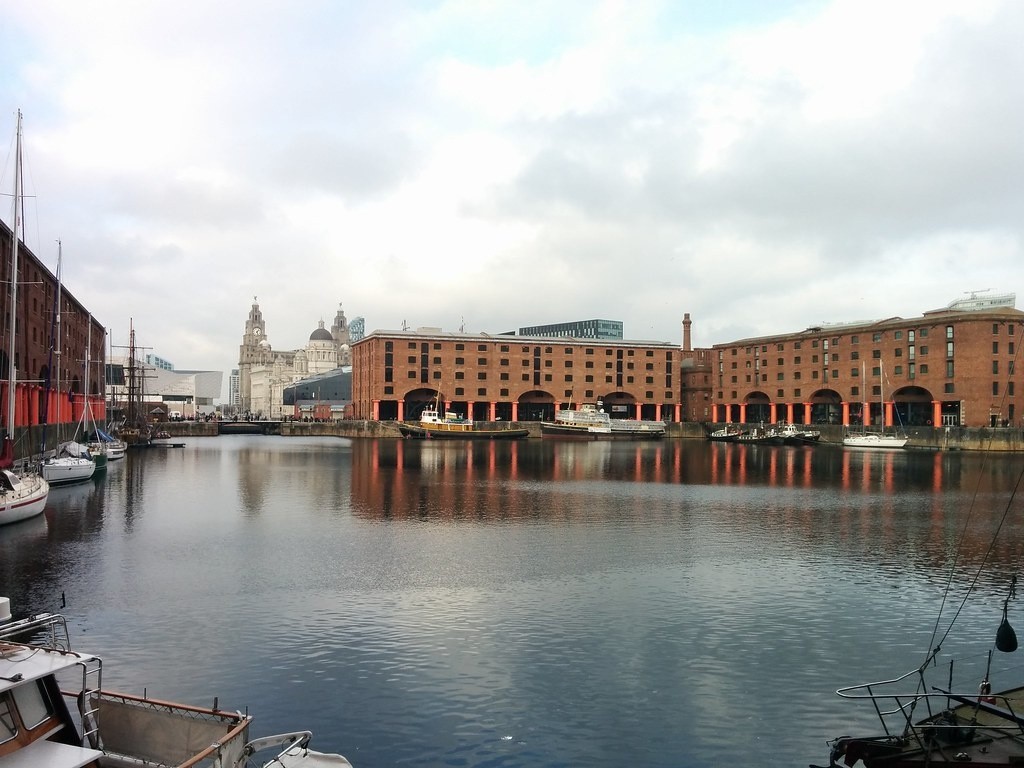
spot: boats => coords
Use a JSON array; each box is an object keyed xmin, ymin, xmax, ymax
[
  {"xmin": 830, "ymin": 325, "xmax": 1024, "ymax": 768},
  {"xmin": 539, "ymin": 401, "xmax": 668, "ymax": 441},
  {"xmin": 398, "ymin": 379, "xmax": 531, "ymax": 441},
  {"xmin": 1, "ymin": 597, "xmax": 353, "ymax": 768},
  {"xmin": 705, "ymin": 418, "xmax": 821, "ymax": 445}
]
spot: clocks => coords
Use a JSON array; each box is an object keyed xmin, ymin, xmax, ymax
[{"xmin": 253, "ymin": 328, "xmax": 262, "ymax": 336}]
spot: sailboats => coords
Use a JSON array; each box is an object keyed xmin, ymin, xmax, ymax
[
  {"xmin": 842, "ymin": 359, "xmax": 909, "ymax": 449},
  {"xmin": 1, "ymin": 108, "xmax": 50, "ymax": 527},
  {"xmin": 71, "ymin": 309, "xmax": 127, "ymax": 469},
  {"xmin": 113, "ymin": 317, "xmax": 159, "ymax": 445},
  {"xmin": 35, "ymin": 240, "xmax": 96, "ymax": 482}
]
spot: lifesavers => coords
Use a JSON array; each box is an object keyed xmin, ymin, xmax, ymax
[{"xmin": 927, "ymin": 419, "xmax": 931, "ymax": 424}]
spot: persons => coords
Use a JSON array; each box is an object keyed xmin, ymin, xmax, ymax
[
  {"xmin": 281, "ymin": 416, "xmax": 325, "ymax": 422},
  {"xmin": 232, "ymin": 412, "xmax": 260, "ymax": 422},
  {"xmin": 206, "ymin": 411, "xmax": 222, "ymax": 422},
  {"xmin": 169, "ymin": 413, "xmax": 194, "ymax": 422}
]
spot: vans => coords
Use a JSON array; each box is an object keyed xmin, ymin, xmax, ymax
[{"xmin": 167, "ymin": 410, "xmax": 181, "ymax": 420}]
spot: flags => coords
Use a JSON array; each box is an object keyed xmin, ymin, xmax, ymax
[{"xmin": 858, "ymin": 409, "xmax": 861, "ymax": 417}]
